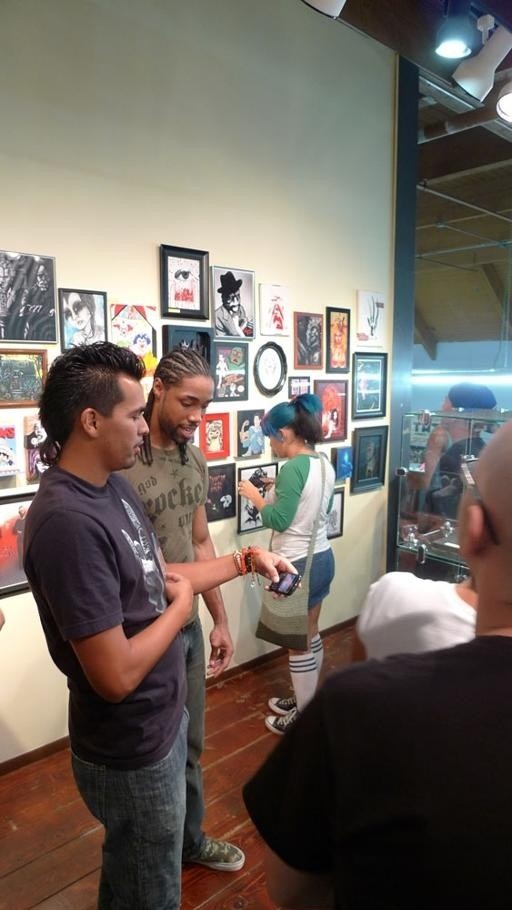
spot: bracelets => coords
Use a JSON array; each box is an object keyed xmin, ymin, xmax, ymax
[{"xmin": 233, "ymin": 546, "xmax": 262, "ymax": 589}]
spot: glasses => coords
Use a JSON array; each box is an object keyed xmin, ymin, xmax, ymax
[{"xmin": 296, "ymin": 0, "xmax": 512, "ymax": 125}]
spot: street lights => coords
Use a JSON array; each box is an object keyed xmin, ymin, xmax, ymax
[{"xmin": 253, "ymin": 578, "xmax": 313, "ymax": 650}]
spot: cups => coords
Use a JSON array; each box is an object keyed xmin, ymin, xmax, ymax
[{"xmin": 460, "ymin": 459, "xmax": 501, "ymax": 546}]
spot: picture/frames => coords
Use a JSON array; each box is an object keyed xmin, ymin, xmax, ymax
[
  {"xmin": 160, "ymin": 245, "xmax": 210, "ymax": 321},
  {"xmin": 324, "ymin": 486, "xmax": 343, "ymax": 539},
  {"xmin": 238, "ymin": 409, "xmax": 265, "ymax": 461},
  {"xmin": 254, "ymin": 341, "xmax": 288, "ymax": 397},
  {"xmin": 260, "ymin": 284, "xmax": 290, "ymax": 337},
  {"xmin": 356, "ymin": 290, "xmax": 386, "ymax": 347},
  {"xmin": 351, "ymin": 352, "xmax": 386, "ymax": 420},
  {"xmin": 199, "ymin": 412, "xmax": 232, "ymax": 462},
  {"xmin": 2, "ymin": 493, "xmax": 36, "ymax": 594},
  {"xmin": 2, "ymin": 251, "xmax": 57, "ymax": 344},
  {"xmin": 333, "ymin": 448, "xmax": 353, "ymax": 480},
  {"xmin": 293, "ymin": 311, "xmax": 324, "ymax": 370},
  {"xmin": 351, "ymin": 426, "xmax": 389, "ymax": 494},
  {"xmin": 2, "ymin": 349, "xmax": 47, "ymax": 407},
  {"xmin": 325, "ymin": 307, "xmax": 351, "ymax": 373},
  {"xmin": 235, "ymin": 462, "xmax": 280, "ymax": 533},
  {"xmin": 20, "ymin": 412, "xmax": 50, "ymax": 484},
  {"xmin": 211, "ymin": 265, "xmax": 255, "ymax": 339},
  {"xmin": 211, "ymin": 341, "xmax": 250, "ymax": 402},
  {"xmin": 206, "ymin": 464, "xmax": 237, "ymax": 523},
  {"xmin": 312, "ymin": 379, "xmax": 347, "ymax": 442},
  {"xmin": 2, "ymin": 424, "xmax": 22, "ymax": 493},
  {"xmin": 288, "ymin": 377, "xmax": 310, "ymax": 398},
  {"xmin": 163, "ymin": 325, "xmax": 214, "ymax": 372},
  {"xmin": 111, "ymin": 303, "xmax": 161, "ymax": 378},
  {"xmin": 58, "ymin": 288, "xmax": 109, "ymax": 354}
]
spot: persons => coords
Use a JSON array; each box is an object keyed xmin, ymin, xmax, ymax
[
  {"xmin": 351, "ymin": 571, "xmax": 480, "ymax": 662},
  {"xmin": 237, "ymin": 394, "xmax": 337, "ymax": 737},
  {"xmin": 242, "ymin": 423, "xmax": 512, "ymax": 910},
  {"xmin": 406, "ymin": 384, "xmax": 497, "ymax": 535},
  {"xmin": 113, "ymin": 349, "xmax": 245, "ymax": 871},
  {"xmin": 23, "ymin": 343, "xmax": 301, "ymax": 908}
]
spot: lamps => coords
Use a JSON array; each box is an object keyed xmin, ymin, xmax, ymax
[{"xmin": 296, "ymin": 0, "xmax": 512, "ymax": 125}]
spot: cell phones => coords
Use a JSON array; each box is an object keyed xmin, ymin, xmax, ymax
[{"xmin": 251, "ymin": 478, "xmax": 265, "ymax": 488}]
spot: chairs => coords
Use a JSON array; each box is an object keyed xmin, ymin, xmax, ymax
[
  {"xmin": 267, "ymin": 694, "xmax": 299, "ymax": 714},
  {"xmin": 192, "ymin": 835, "xmax": 246, "ymax": 874},
  {"xmin": 264, "ymin": 714, "xmax": 297, "ymax": 737}
]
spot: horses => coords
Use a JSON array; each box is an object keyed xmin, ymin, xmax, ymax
[{"xmin": 270, "ymin": 571, "xmax": 301, "ymax": 596}]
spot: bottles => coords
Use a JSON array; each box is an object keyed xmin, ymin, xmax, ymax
[
  {"xmin": 449, "ymin": 381, "xmax": 497, "ymax": 409},
  {"xmin": 217, "ymin": 270, "xmax": 244, "ymax": 293}
]
